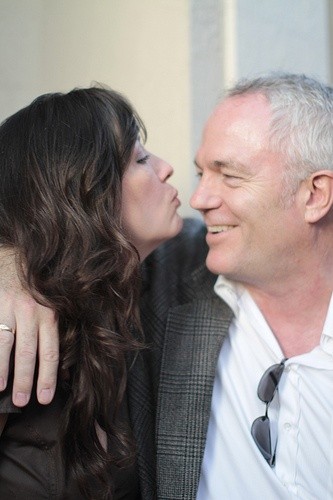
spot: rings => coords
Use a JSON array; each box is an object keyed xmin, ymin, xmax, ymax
[{"xmin": 0, "ymin": 324, "xmax": 13, "ymax": 333}]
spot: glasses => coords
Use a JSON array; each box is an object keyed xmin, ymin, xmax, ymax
[{"xmin": 251, "ymin": 359, "xmax": 287, "ymax": 465}]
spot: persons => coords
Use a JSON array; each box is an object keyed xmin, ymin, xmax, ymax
[
  {"xmin": 9, "ymin": 75, "xmax": 333, "ymax": 500},
  {"xmin": 0, "ymin": 79, "xmax": 184, "ymax": 500}
]
